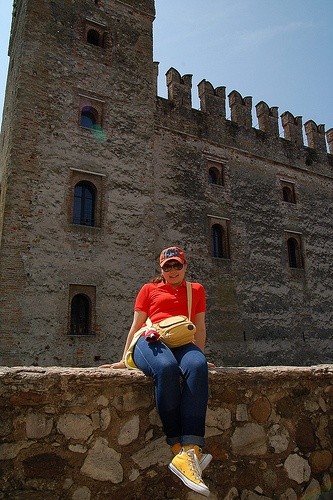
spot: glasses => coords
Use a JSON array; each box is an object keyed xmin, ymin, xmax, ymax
[{"xmin": 160, "ymin": 262, "xmax": 183, "ymax": 272}]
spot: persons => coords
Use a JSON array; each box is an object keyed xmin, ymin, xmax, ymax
[{"xmin": 95, "ymin": 244, "xmax": 217, "ymax": 498}]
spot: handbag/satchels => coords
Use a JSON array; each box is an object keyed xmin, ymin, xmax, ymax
[{"xmin": 146, "ymin": 315, "xmax": 197, "ymax": 348}]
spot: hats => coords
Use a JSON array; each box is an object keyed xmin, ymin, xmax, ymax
[{"xmin": 159, "ymin": 246, "xmax": 185, "ymax": 268}]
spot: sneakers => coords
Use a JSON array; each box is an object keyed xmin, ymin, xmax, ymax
[{"xmin": 168, "ymin": 443, "xmax": 213, "ymax": 497}]
